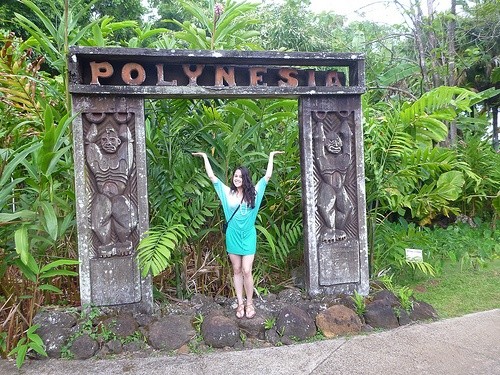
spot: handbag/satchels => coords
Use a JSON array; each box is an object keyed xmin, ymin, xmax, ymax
[{"xmin": 222, "ymin": 223, "xmax": 228, "ymax": 233}]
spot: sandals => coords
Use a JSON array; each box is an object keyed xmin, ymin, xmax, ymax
[
  {"xmin": 245, "ymin": 304, "xmax": 256, "ymax": 318},
  {"xmin": 236, "ymin": 304, "xmax": 244, "ymax": 319}
]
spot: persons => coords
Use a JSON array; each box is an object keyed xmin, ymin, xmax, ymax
[{"xmin": 192, "ymin": 151, "xmax": 285, "ymax": 319}]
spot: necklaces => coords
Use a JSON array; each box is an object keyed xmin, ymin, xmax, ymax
[{"xmin": 237, "ymin": 195, "xmax": 248, "ymax": 215}]
